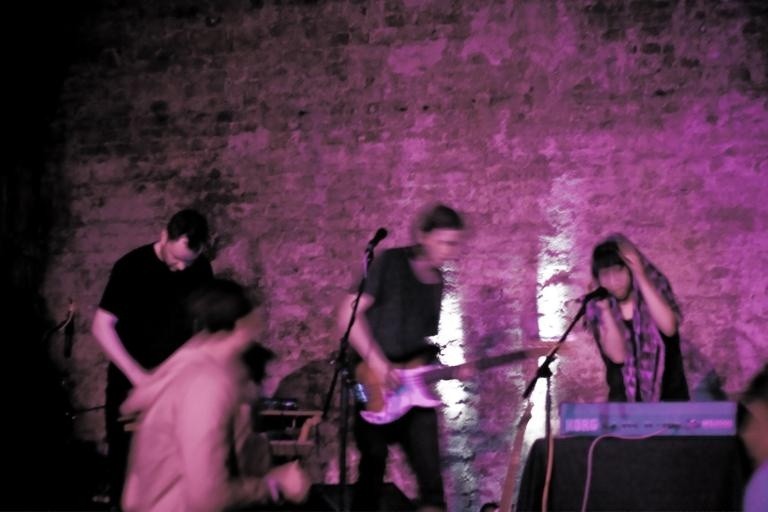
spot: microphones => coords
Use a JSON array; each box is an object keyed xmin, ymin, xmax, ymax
[
  {"xmin": 576, "ymin": 287, "xmax": 607, "ymax": 303},
  {"xmin": 63, "ymin": 302, "xmax": 74, "ymax": 358},
  {"xmin": 365, "ymin": 228, "xmax": 387, "ymax": 252}
]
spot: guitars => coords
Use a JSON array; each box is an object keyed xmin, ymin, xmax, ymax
[{"xmin": 356, "ymin": 340, "xmax": 569, "ymax": 424}]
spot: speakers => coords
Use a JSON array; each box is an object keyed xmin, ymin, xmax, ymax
[{"xmin": 301, "ymin": 483, "xmax": 416, "ymax": 512}]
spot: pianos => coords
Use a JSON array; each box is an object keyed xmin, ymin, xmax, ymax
[
  {"xmin": 117, "ymin": 397, "xmax": 299, "ymax": 423},
  {"xmin": 559, "ymin": 401, "xmax": 737, "ymax": 439}
]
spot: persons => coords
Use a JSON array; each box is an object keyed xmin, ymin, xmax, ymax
[
  {"xmin": 340, "ymin": 202, "xmax": 479, "ymax": 511},
  {"xmin": 737, "ymin": 359, "xmax": 767, "ymax": 511},
  {"xmin": 583, "ymin": 234, "xmax": 687, "ymax": 400},
  {"xmin": 88, "ymin": 206, "xmax": 213, "ymax": 512},
  {"xmin": 117, "ymin": 269, "xmax": 311, "ymax": 510}
]
